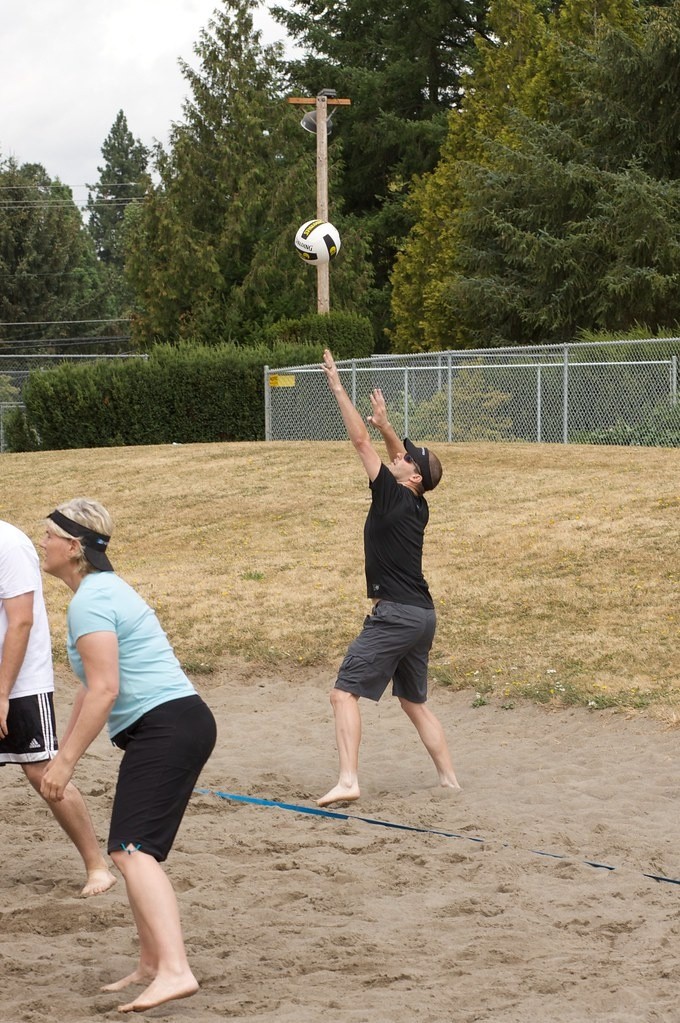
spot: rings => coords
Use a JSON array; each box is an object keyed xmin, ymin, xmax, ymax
[{"xmin": 327, "ymin": 365, "xmax": 332, "ymax": 369}]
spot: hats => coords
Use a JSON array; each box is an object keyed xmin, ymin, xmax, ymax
[
  {"xmin": 46, "ymin": 509, "xmax": 115, "ymax": 571},
  {"xmin": 403, "ymin": 438, "xmax": 433, "ymax": 490}
]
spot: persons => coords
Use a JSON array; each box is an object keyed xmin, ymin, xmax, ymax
[
  {"xmin": 40, "ymin": 500, "xmax": 217, "ymax": 1012},
  {"xmin": 316, "ymin": 350, "xmax": 461, "ymax": 807},
  {"xmin": 0, "ymin": 519, "xmax": 117, "ymax": 898}
]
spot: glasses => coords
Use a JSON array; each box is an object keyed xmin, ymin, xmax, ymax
[{"xmin": 403, "ymin": 453, "xmax": 421, "ymax": 475}]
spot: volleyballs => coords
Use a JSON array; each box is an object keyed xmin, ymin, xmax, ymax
[{"xmin": 294, "ymin": 218, "xmax": 341, "ymax": 267}]
